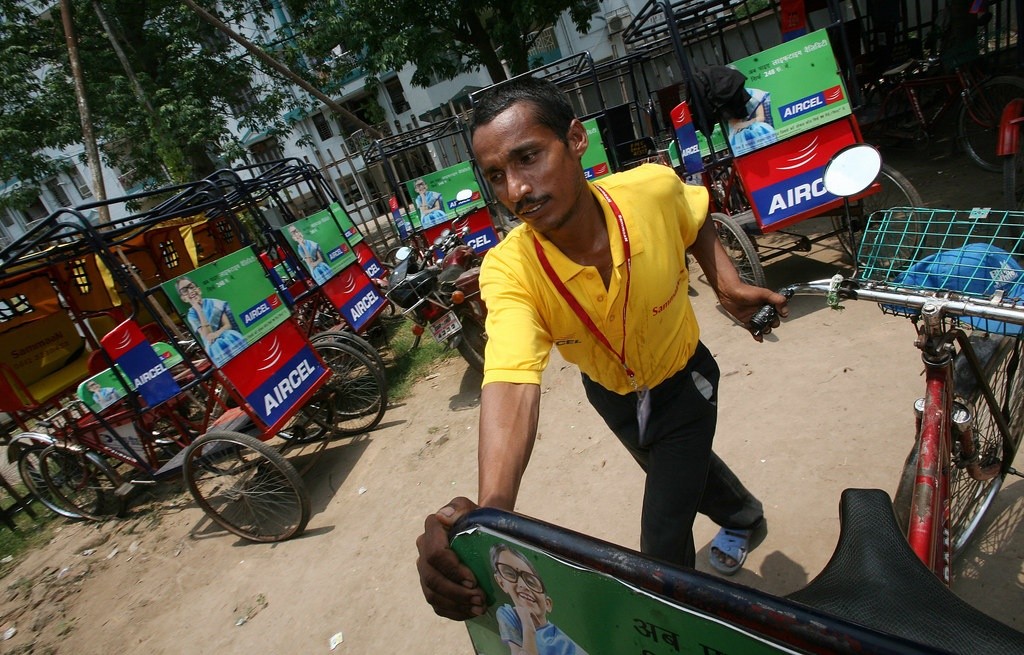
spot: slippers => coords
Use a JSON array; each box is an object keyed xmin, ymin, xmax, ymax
[{"xmin": 710, "ymin": 514, "xmax": 764, "ymax": 573}]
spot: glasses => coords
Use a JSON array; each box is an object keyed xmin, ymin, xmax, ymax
[
  {"xmin": 179, "ymin": 282, "xmax": 197, "ymax": 296},
  {"xmin": 416, "ymin": 182, "xmax": 426, "ymax": 188},
  {"xmin": 290, "ymin": 229, "xmax": 299, "ymax": 235}
]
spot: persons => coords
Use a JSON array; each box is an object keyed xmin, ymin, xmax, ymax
[
  {"xmin": 413, "ymin": 178, "xmax": 448, "ymax": 229},
  {"xmin": 87, "ymin": 380, "xmax": 121, "ymax": 409},
  {"xmin": 175, "ymin": 276, "xmax": 249, "ymax": 369},
  {"xmin": 489, "ymin": 542, "xmax": 588, "ymax": 655},
  {"xmin": 288, "ymin": 225, "xmax": 335, "ymax": 286},
  {"xmin": 415, "ymin": 73, "xmax": 791, "ymax": 622},
  {"xmin": 722, "ymin": 64, "xmax": 779, "ymax": 158}
]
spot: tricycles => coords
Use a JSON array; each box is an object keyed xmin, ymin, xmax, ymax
[{"xmin": 0, "ymin": 11, "xmax": 1023, "ymax": 654}]
